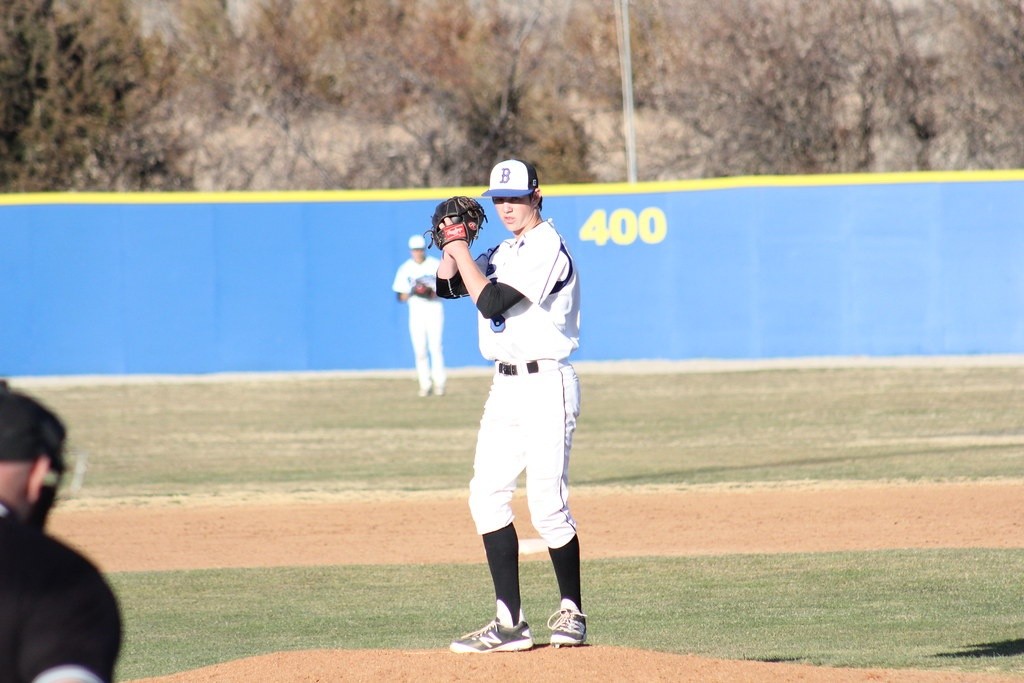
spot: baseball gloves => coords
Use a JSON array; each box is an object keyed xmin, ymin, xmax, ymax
[
  {"xmin": 412, "ymin": 279, "xmax": 435, "ymax": 300},
  {"xmin": 422, "ymin": 195, "xmax": 488, "ymax": 251}
]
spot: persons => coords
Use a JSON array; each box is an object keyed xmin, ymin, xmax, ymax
[
  {"xmin": 0, "ymin": 380, "xmax": 123, "ymax": 683},
  {"xmin": 392, "ymin": 234, "xmax": 447, "ymax": 397},
  {"xmin": 424, "ymin": 159, "xmax": 588, "ymax": 653}
]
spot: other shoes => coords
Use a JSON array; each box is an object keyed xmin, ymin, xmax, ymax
[
  {"xmin": 434, "ymin": 386, "xmax": 444, "ymax": 395},
  {"xmin": 418, "ymin": 389, "xmax": 431, "ymax": 397}
]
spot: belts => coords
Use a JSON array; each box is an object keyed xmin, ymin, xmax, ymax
[{"xmin": 495, "ymin": 359, "xmax": 538, "ymax": 375}]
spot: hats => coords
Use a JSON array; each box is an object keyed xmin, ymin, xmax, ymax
[
  {"xmin": 481, "ymin": 159, "xmax": 538, "ymax": 197},
  {"xmin": 408, "ymin": 235, "xmax": 426, "ymax": 249},
  {"xmin": 0, "ymin": 393, "xmax": 65, "ymax": 460}
]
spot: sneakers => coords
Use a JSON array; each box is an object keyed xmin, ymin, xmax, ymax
[
  {"xmin": 547, "ymin": 598, "xmax": 587, "ymax": 649},
  {"xmin": 450, "ymin": 599, "xmax": 534, "ymax": 654}
]
